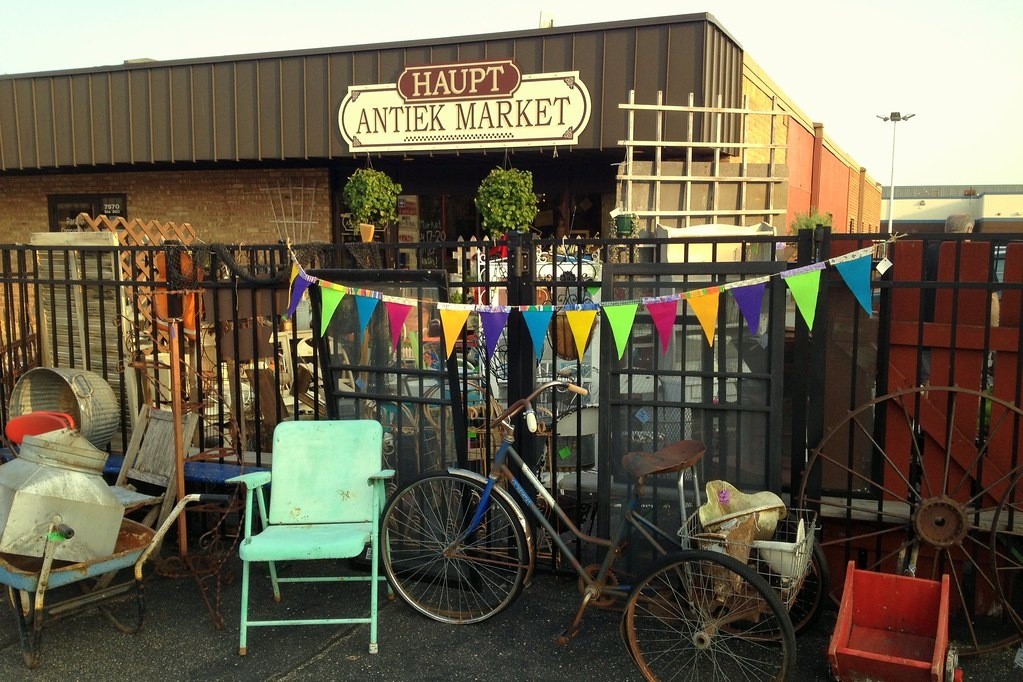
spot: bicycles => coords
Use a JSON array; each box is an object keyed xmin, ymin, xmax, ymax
[{"xmin": 379, "ymin": 379, "xmax": 795, "ymax": 682}]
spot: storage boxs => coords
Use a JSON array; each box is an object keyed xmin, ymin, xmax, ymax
[{"xmin": 825, "ymin": 558, "xmax": 948, "ymax": 682}]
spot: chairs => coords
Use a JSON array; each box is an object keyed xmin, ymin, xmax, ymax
[
  {"xmin": 226, "ymin": 417, "xmax": 395, "ymax": 657},
  {"xmin": 425, "ymin": 381, "xmax": 512, "ymax": 488},
  {"xmin": 110, "ymin": 404, "xmax": 198, "ymax": 569}
]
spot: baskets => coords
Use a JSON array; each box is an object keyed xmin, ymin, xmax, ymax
[{"xmin": 677, "ymin": 500, "xmax": 817, "ymax": 615}]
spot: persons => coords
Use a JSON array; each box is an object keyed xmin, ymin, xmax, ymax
[{"xmin": 943, "ymin": 213, "xmax": 998, "ymax": 367}]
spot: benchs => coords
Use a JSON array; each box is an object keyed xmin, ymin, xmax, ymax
[{"xmin": 104, "ymin": 453, "xmax": 273, "ymax": 488}]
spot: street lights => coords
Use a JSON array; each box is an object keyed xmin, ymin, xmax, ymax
[{"xmin": 878, "ymin": 112, "xmax": 918, "ymax": 240}]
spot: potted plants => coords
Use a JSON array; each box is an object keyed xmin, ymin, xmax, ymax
[{"xmin": 608, "ymin": 211, "xmax": 639, "ymax": 281}]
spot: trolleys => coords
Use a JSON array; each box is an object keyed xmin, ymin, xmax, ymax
[{"xmin": 13, "ymin": 493, "xmax": 202, "ymax": 671}]
